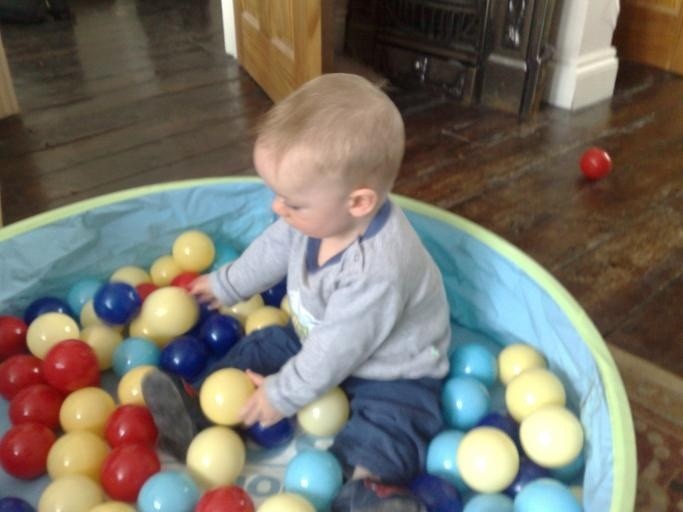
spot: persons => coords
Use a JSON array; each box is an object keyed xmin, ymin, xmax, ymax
[{"xmin": 142, "ymin": 73, "xmax": 452, "ymax": 512}]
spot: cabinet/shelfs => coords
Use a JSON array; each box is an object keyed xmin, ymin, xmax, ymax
[{"xmin": 344, "ymin": 0, "xmax": 557, "ymax": 120}]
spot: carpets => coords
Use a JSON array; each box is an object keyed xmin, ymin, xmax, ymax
[{"xmin": 604, "ymin": 342, "xmax": 683, "ymax": 512}]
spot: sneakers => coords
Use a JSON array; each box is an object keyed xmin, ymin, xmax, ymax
[
  {"xmin": 141, "ymin": 369, "xmax": 209, "ymax": 462},
  {"xmin": 332, "ymin": 478, "xmax": 428, "ymax": 512}
]
